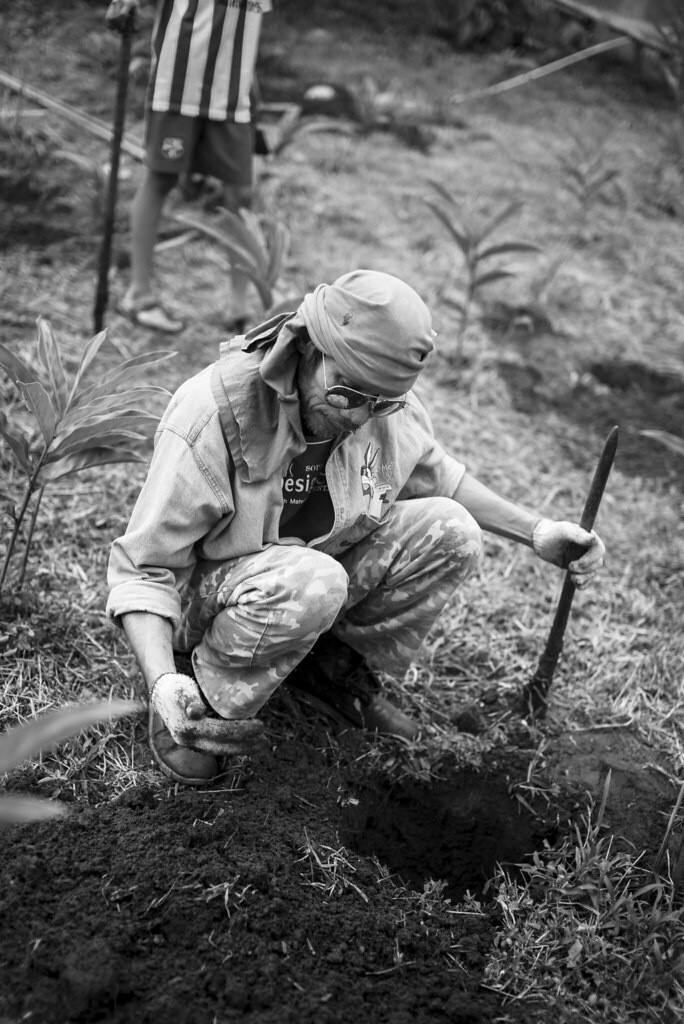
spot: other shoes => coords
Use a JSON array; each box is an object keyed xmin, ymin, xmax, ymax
[
  {"xmin": 220, "ymin": 293, "xmax": 257, "ymax": 333},
  {"xmin": 113, "ymin": 287, "xmax": 184, "ymax": 336}
]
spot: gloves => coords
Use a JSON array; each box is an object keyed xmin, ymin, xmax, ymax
[
  {"xmin": 532, "ymin": 517, "xmax": 607, "ymax": 591},
  {"xmin": 150, "ymin": 672, "xmax": 264, "ymax": 756}
]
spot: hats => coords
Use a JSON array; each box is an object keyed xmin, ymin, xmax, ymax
[{"xmin": 211, "ymin": 269, "xmax": 436, "ymax": 484}]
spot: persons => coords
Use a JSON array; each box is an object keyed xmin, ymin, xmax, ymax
[
  {"xmin": 107, "ymin": 270, "xmax": 605, "ymax": 785},
  {"xmin": 106, "ymin": 0, "xmax": 272, "ymax": 337}
]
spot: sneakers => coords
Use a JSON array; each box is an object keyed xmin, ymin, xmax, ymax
[
  {"xmin": 148, "ymin": 673, "xmax": 218, "ymax": 784},
  {"xmin": 290, "ymin": 652, "xmax": 423, "ymax": 739}
]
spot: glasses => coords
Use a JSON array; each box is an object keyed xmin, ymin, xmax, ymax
[{"xmin": 322, "ymin": 344, "xmax": 407, "ymax": 419}]
